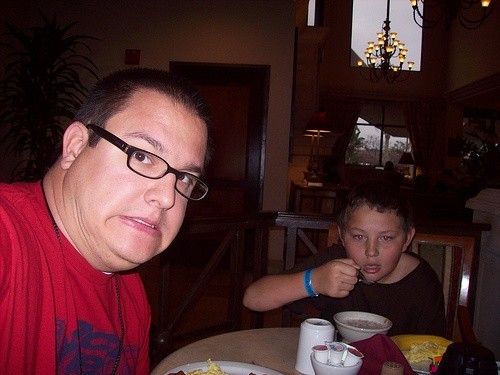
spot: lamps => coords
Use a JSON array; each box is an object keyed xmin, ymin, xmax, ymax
[
  {"xmin": 357, "ymin": 0, "xmax": 414, "ymax": 84},
  {"xmin": 305, "ymin": 112, "xmax": 333, "ymax": 182},
  {"xmin": 410, "ymin": 0, "xmax": 494, "ymax": 32},
  {"xmin": 399, "ymin": 152, "xmax": 416, "ymax": 175},
  {"xmin": 301, "ymin": 119, "xmax": 324, "ymax": 179}
]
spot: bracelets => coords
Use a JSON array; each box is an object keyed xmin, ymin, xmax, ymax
[{"xmin": 304, "ymin": 269, "xmax": 317, "ymax": 297}]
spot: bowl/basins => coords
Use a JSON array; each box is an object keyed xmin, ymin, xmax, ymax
[
  {"xmin": 334, "ymin": 311, "xmax": 393, "ymax": 343},
  {"xmin": 311, "ymin": 352, "xmax": 363, "ymax": 375}
]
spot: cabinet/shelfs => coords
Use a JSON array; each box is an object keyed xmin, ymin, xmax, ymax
[{"xmin": 288, "ymin": 180, "xmax": 352, "ymax": 258}]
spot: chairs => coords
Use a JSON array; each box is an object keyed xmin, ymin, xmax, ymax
[
  {"xmin": 327, "ymin": 223, "xmax": 481, "ymax": 345},
  {"xmin": 151, "ymin": 212, "xmax": 280, "ymax": 364}
]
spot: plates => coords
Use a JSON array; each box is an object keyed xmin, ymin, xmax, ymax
[
  {"xmin": 389, "ymin": 333, "xmax": 455, "ymax": 375},
  {"xmin": 162, "ymin": 361, "xmax": 285, "ymax": 375}
]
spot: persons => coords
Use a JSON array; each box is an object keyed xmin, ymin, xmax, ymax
[
  {"xmin": 0, "ymin": 66, "xmax": 217, "ymax": 375},
  {"xmin": 242, "ymin": 181, "xmax": 447, "ymax": 343}
]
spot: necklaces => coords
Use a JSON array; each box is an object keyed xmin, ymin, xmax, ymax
[{"xmin": 49, "ymin": 215, "xmax": 124, "ymax": 375}]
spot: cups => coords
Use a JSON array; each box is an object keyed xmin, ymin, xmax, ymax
[
  {"xmin": 381, "ymin": 362, "xmax": 404, "ymax": 375},
  {"xmin": 312, "ymin": 344, "xmax": 328, "ymax": 364},
  {"xmin": 343, "ymin": 347, "xmax": 364, "ymax": 366},
  {"xmin": 294, "ymin": 318, "xmax": 335, "ymax": 375},
  {"xmin": 328, "ymin": 342, "xmax": 347, "ymax": 365}
]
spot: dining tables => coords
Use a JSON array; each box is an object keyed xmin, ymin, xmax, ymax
[{"xmin": 150, "ymin": 327, "xmax": 300, "ymax": 375}]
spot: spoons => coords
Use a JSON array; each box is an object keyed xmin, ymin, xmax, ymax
[{"xmin": 357, "ymin": 270, "xmax": 374, "ymax": 284}]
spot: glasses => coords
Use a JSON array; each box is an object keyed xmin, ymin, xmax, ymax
[{"xmin": 84, "ymin": 124, "xmax": 209, "ymax": 202}]
